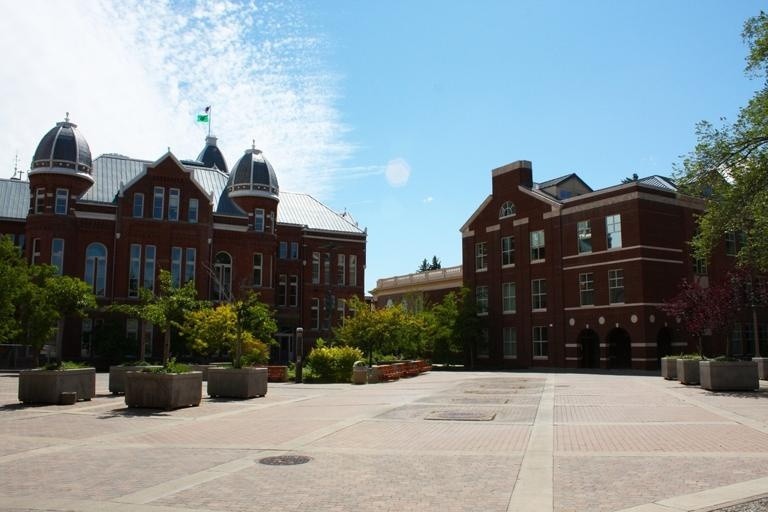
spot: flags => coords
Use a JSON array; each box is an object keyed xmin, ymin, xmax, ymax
[{"xmin": 196, "ymin": 115, "xmax": 208, "ymax": 123}]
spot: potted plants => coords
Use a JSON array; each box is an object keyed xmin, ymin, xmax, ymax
[
  {"xmin": 200, "ymin": 262, "xmax": 269, "ymax": 400},
  {"xmin": 10, "ymin": 263, "xmax": 102, "ymax": 404},
  {"xmin": 660, "ymin": 270, "xmax": 760, "ymax": 392},
  {"xmin": 124, "ymin": 270, "xmax": 203, "ymax": 412},
  {"xmin": 101, "ymin": 287, "xmax": 159, "ymax": 396}
]
